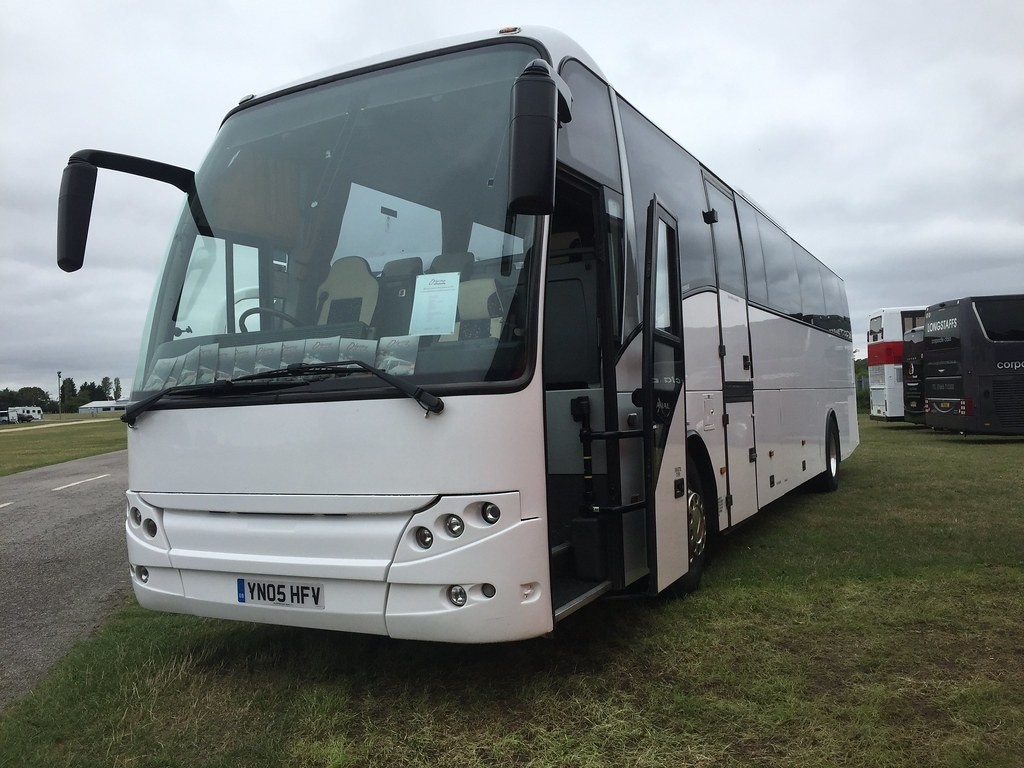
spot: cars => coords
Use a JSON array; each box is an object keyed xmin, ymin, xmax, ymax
[{"xmin": 0, "ymin": 410, "xmax": 34, "ymax": 426}]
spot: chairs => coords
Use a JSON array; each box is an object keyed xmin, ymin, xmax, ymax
[
  {"xmin": 523, "ymin": 231, "xmax": 583, "ymax": 268},
  {"xmin": 317, "ymin": 251, "xmax": 507, "ymax": 341}
]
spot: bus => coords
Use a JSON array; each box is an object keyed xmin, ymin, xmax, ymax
[
  {"xmin": 866, "ymin": 303, "xmax": 931, "ymax": 423},
  {"xmin": 56, "ymin": 22, "xmax": 862, "ymax": 644},
  {"xmin": 903, "ymin": 325, "xmax": 925, "ymax": 426},
  {"xmin": 919, "ymin": 294, "xmax": 1024, "ymax": 436}
]
